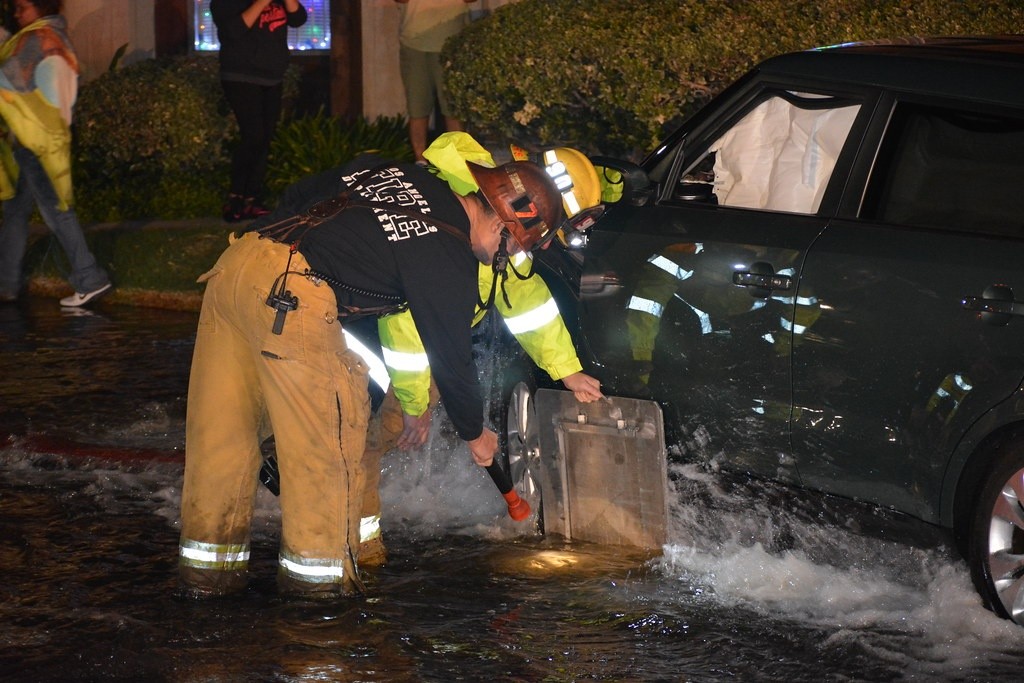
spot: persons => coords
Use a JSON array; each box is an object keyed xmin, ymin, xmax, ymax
[
  {"xmin": 621, "ymin": 244, "xmax": 820, "ymax": 394},
  {"xmin": 261, "ymin": 130, "xmax": 598, "ymax": 564},
  {"xmin": 206, "ymin": 0, "xmax": 308, "ymax": 222},
  {"xmin": 0, "ymin": 0, "xmax": 111, "ymax": 308},
  {"xmin": 174, "ymin": 156, "xmax": 560, "ymax": 607},
  {"xmin": 393, "ymin": 0, "xmax": 478, "ymax": 163}
]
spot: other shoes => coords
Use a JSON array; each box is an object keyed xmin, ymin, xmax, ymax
[{"xmin": 222, "ymin": 194, "xmax": 272, "ymax": 220}]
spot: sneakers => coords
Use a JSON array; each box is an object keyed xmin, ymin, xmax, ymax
[{"xmin": 60, "ymin": 280, "xmax": 111, "ymax": 306}]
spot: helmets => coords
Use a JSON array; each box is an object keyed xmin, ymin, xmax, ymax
[
  {"xmin": 464, "ymin": 159, "xmax": 563, "ymax": 255},
  {"xmin": 509, "ymin": 142, "xmax": 603, "ymax": 249}
]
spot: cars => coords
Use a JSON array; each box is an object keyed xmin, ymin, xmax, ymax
[{"xmin": 468, "ymin": 34, "xmax": 1024, "ymax": 625}]
